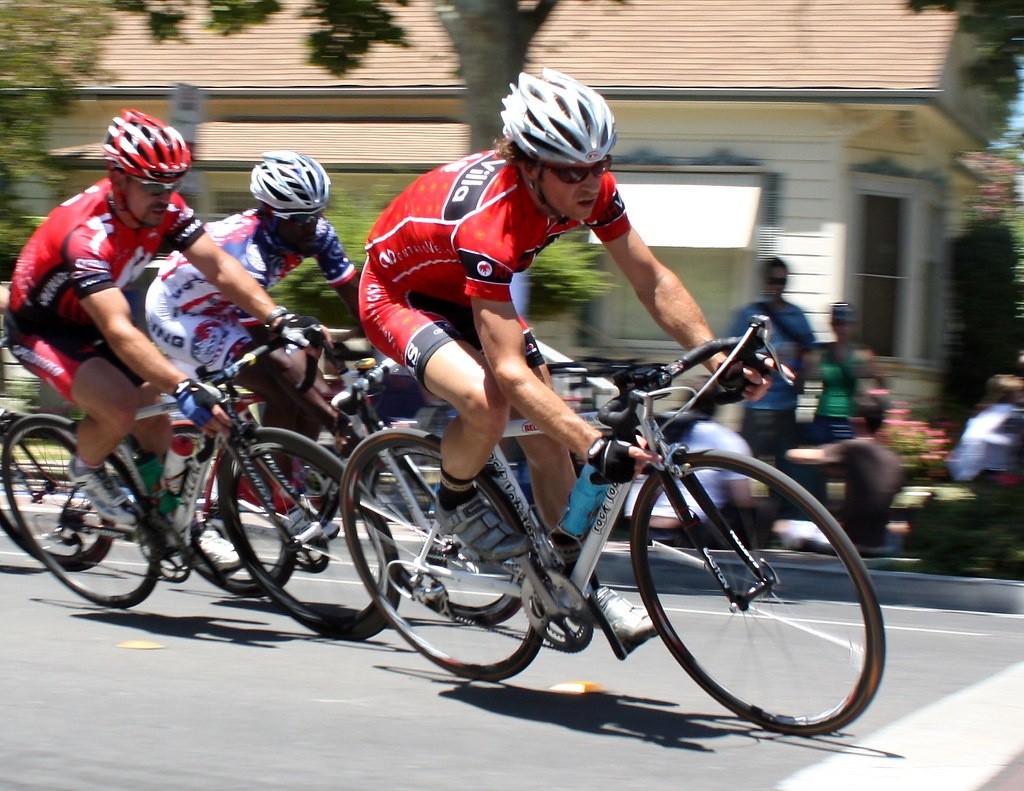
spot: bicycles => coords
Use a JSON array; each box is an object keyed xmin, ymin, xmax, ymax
[
  {"xmin": 0, "ymin": 321, "xmax": 526, "ymax": 642},
  {"xmin": 338, "ymin": 313, "xmax": 887, "ymax": 738}
]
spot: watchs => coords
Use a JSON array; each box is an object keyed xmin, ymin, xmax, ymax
[{"xmin": 264, "ymin": 306, "xmax": 288, "ymax": 325}]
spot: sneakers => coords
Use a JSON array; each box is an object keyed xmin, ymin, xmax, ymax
[
  {"xmin": 194, "ymin": 527, "xmax": 240, "ymax": 571},
  {"xmin": 584, "ymin": 586, "xmax": 659, "ymax": 639},
  {"xmin": 67, "ymin": 457, "xmax": 141, "ymax": 526},
  {"xmin": 433, "ymin": 487, "xmax": 535, "ymax": 561},
  {"xmin": 285, "ymin": 505, "xmax": 341, "ymax": 544}
]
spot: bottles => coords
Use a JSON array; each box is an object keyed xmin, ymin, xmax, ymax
[
  {"xmin": 279, "ymin": 451, "xmax": 306, "ymax": 495},
  {"xmin": 561, "ymin": 459, "xmax": 608, "ymax": 537},
  {"xmin": 134, "ymin": 452, "xmax": 180, "ymax": 513},
  {"xmin": 163, "ymin": 436, "xmax": 194, "ymax": 493}
]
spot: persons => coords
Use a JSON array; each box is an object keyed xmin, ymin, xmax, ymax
[
  {"xmin": 3, "ymin": 107, "xmax": 332, "ymax": 572},
  {"xmin": 143, "ymin": 150, "xmax": 363, "ymax": 543},
  {"xmin": 358, "ymin": 71, "xmax": 794, "ymax": 657},
  {"xmin": 720, "ymin": 258, "xmax": 900, "ymax": 549},
  {"xmin": 624, "ymin": 382, "xmax": 765, "ymax": 548},
  {"xmin": 948, "ymin": 374, "xmax": 1024, "ymax": 481}
]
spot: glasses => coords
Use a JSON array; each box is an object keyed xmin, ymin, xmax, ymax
[
  {"xmin": 546, "ymin": 154, "xmax": 615, "ymax": 184},
  {"xmin": 270, "ymin": 205, "xmax": 327, "ymax": 223},
  {"xmin": 129, "ymin": 174, "xmax": 184, "ymax": 195}
]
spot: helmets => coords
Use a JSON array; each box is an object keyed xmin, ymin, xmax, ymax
[
  {"xmin": 501, "ymin": 68, "xmax": 620, "ymax": 166},
  {"xmin": 249, "ymin": 151, "xmax": 330, "ymax": 214},
  {"xmin": 102, "ymin": 107, "xmax": 191, "ymax": 181}
]
